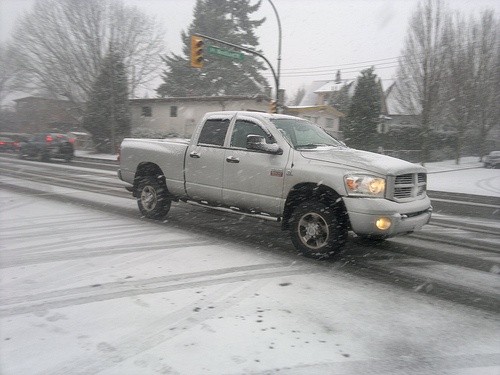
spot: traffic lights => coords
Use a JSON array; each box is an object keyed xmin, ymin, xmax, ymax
[{"xmin": 191, "ymin": 35, "xmax": 205, "ymax": 68}]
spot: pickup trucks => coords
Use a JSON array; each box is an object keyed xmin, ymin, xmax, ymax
[{"xmin": 118, "ymin": 111, "xmax": 433, "ymax": 261}]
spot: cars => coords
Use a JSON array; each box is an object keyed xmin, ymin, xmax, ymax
[{"xmin": 0, "ymin": 133, "xmax": 74, "ymax": 162}]
